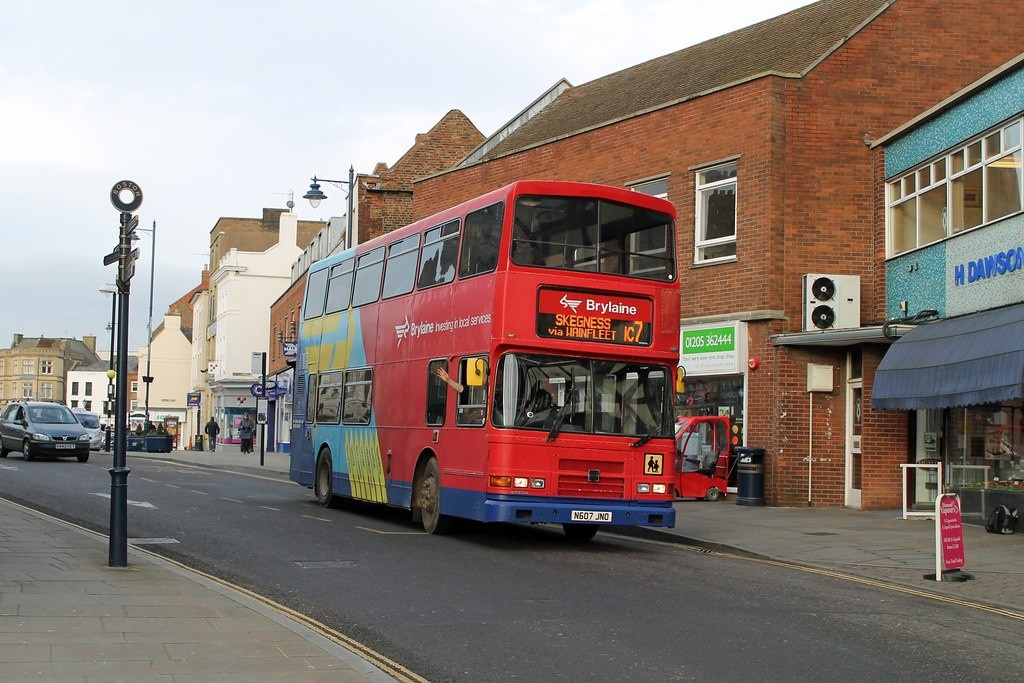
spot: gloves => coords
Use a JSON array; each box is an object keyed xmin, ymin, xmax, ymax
[
  {"xmin": 243, "ymin": 426, "xmax": 245, "ymax": 429},
  {"xmin": 247, "ymin": 426, "xmax": 250, "ymax": 430}
]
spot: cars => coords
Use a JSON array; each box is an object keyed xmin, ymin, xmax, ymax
[
  {"xmin": 67, "ymin": 406, "xmax": 105, "ymax": 452},
  {"xmin": 0, "ymin": 398, "xmax": 91, "ymax": 462}
]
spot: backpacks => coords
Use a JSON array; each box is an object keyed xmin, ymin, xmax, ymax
[{"xmin": 985, "ymin": 504, "xmax": 1019, "ymax": 533}]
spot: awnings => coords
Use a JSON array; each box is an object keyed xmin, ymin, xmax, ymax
[{"xmin": 871, "ymin": 304, "xmax": 1024, "ymax": 410}]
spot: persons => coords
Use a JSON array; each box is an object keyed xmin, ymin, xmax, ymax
[
  {"xmin": 239, "ymin": 412, "xmax": 254, "ymax": 454},
  {"xmin": 136, "ymin": 421, "xmax": 163, "ymax": 432},
  {"xmin": 437, "ymin": 367, "xmax": 504, "ymax": 414},
  {"xmin": 205, "ymin": 417, "xmax": 220, "ymax": 452}
]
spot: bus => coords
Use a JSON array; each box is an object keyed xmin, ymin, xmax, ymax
[{"xmin": 289, "ymin": 179, "xmax": 683, "ymax": 535}]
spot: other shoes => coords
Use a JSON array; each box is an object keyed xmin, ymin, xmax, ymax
[
  {"xmin": 244, "ymin": 450, "xmax": 246, "ymax": 454},
  {"xmin": 212, "ymin": 449, "xmax": 215, "ymax": 451},
  {"xmin": 248, "ymin": 450, "xmax": 250, "ymax": 453}
]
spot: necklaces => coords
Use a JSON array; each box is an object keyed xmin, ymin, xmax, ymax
[{"xmin": 494, "ymin": 395, "xmax": 501, "ymax": 408}]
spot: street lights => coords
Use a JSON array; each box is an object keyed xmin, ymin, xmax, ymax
[
  {"xmin": 99, "ymin": 288, "xmax": 117, "ymax": 370},
  {"xmin": 303, "ymin": 164, "xmax": 354, "ymax": 251}
]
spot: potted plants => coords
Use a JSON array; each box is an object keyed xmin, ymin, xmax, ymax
[
  {"xmin": 147, "ymin": 422, "xmax": 173, "ymax": 453},
  {"xmin": 127, "ymin": 423, "xmax": 145, "ymax": 451}
]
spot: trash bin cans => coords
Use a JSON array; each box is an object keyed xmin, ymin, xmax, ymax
[
  {"xmin": 194, "ymin": 434, "xmax": 203, "ymax": 451},
  {"xmin": 735, "ymin": 446, "xmax": 766, "ymax": 507}
]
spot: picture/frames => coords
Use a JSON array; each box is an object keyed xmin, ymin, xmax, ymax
[{"xmin": 964, "ymin": 189, "xmax": 981, "ymax": 207}]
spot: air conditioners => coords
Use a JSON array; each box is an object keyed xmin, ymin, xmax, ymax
[{"xmin": 802, "ymin": 273, "xmax": 861, "ymax": 333}]
